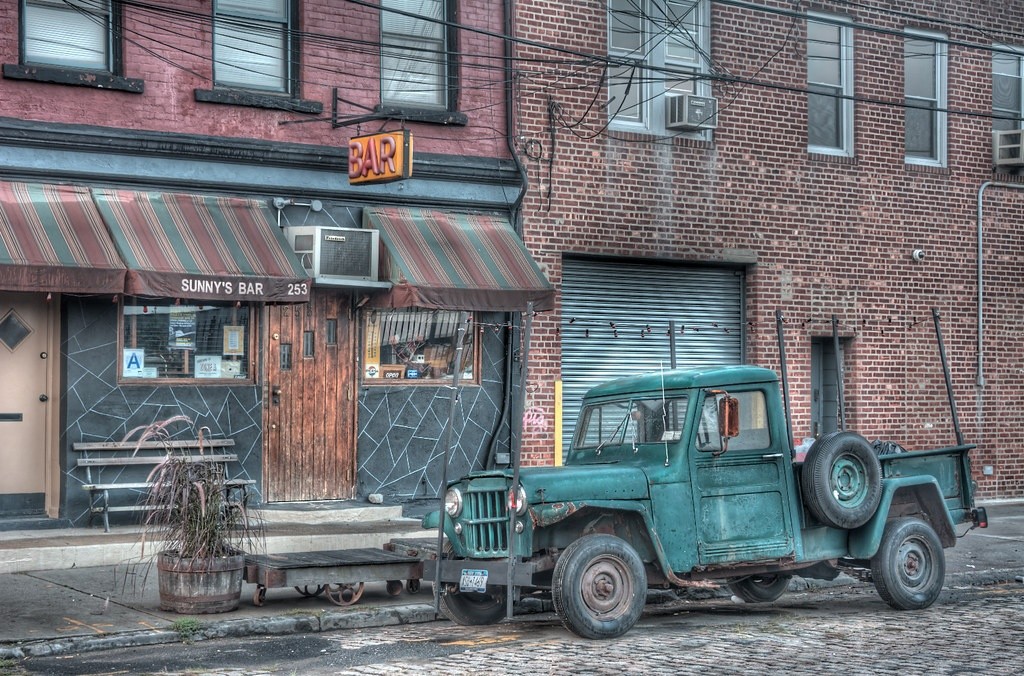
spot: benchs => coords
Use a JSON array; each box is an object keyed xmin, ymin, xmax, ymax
[{"xmin": 72, "ymin": 438, "xmax": 256, "ymax": 533}]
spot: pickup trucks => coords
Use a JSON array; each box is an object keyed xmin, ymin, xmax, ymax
[{"xmin": 423, "ymin": 363, "xmax": 988, "ymax": 641}]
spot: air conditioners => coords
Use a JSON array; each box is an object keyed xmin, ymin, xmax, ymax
[
  {"xmin": 991, "ymin": 128, "xmax": 1024, "ymax": 169},
  {"xmin": 283, "ymin": 225, "xmax": 380, "ymax": 281},
  {"xmin": 665, "ymin": 95, "xmax": 718, "ymax": 133}
]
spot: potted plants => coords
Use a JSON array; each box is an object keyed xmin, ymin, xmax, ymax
[{"xmin": 101, "ymin": 416, "xmax": 270, "ymax": 616}]
